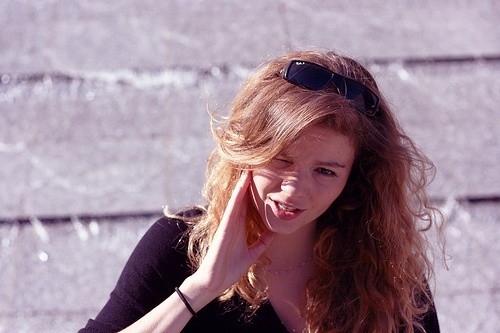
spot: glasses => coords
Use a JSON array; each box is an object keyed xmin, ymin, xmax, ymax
[{"xmin": 277, "ymin": 60, "xmax": 381, "ymax": 117}]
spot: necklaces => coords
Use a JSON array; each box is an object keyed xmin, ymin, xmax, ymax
[{"xmin": 258, "ymin": 255, "xmax": 312, "ymax": 275}]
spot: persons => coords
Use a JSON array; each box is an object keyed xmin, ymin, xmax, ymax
[{"xmin": 78, "ymin": 50, "xmax": 451, "ymax": 333}]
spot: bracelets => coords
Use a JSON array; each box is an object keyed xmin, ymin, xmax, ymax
[{"xmin": 175, "ymin": 285, "xmax": 197, "ymax": 317}]
